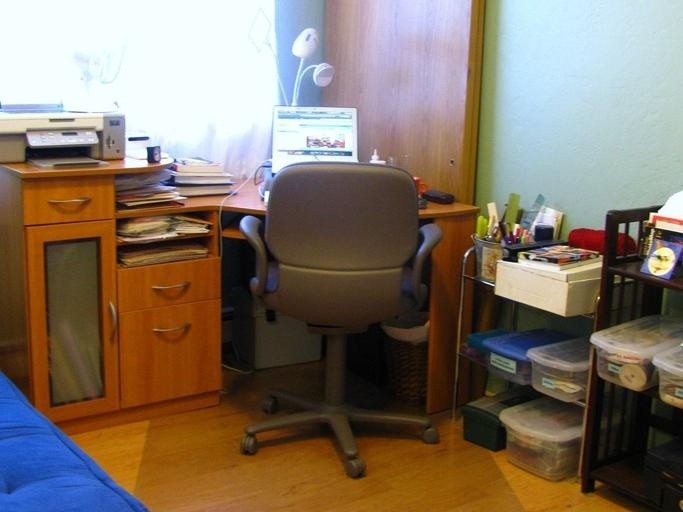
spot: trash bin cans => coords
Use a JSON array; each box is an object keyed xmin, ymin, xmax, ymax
[{"xmin": 381, "ymin": 309, "xmax": 429, "ymax": 406}]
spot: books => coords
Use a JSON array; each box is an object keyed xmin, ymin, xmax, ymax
[
  {"xmin": 163, "ymin": 156, "xmax": 237, "ymax": 197},
  {"xmin": 118, "ymin": 214, "xmax": 211, "ymax": 268},
  {"xmin": 529, "ymin": 207, "xmax": 564, "ymax": 240},
  {"xmin": 115, "ymin": 171, "xmax": 187, "ymax": 211},
  {"xmin": 518, "ymin": 244, "xmax": 604, "ymax": 272}
]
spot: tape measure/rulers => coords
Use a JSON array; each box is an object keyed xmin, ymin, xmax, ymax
[{"xmin": 505, "ymin": 193, "xmax": 520, "ymax": 233}]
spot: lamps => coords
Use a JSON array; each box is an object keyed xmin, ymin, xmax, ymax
[{"xmin": 254, "ymin": 29, "xmax": 336, "ymax": 109}]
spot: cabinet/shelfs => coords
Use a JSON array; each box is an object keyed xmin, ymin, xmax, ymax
[
  {"xmin": 451, "ymin": 226, "xmax": 645, "ymax": 503},
  {"xmin": 580, "ymin": 202, "xmax": 682, "ymax": 511}
]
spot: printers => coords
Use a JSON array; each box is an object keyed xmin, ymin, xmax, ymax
[{"xmin": 0, "ymin": 105, "xmax": 125, "ymax": 169}]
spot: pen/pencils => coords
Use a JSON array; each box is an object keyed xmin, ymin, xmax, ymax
[{"xmin": 476, "ymin": 202, "xmax": 530, "ymax": 244}]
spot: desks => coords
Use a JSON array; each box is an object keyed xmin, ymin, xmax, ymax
[{"xmin": 0, "ymin": 151, "xmax": 481, "ymax": 435}]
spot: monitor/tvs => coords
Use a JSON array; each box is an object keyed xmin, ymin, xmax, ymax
[{"xmin": 271, "ymin": 105, "xmax": 358, "ymax": 175}]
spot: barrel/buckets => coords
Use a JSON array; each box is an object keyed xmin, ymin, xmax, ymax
[{"xmin": 470, "ymin": 231, "xmax": 507, "ymax": 283}]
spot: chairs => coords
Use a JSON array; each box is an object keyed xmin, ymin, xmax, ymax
[{"xmin": 238, "ymin": 164, "xmax": 448, "ymax": 483}]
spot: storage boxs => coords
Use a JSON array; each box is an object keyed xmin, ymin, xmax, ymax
[
  {"xmin": 497, "ymin": 397, "xmax": 581, "ymax": 483},
  {"xmin": 588, "ymin": 311, "xmax": 681, "ymax": 394},
  {"xmin": 466, "ymin": 388, "xmax": 523, "ymax": 454},
  {"xmin": 523, "ymin": 336, "xmax": 592, "ymax": 404},
  {"xmin": 649, "ymin": 342, "xmax": 682, "ymax": 411},
  {"xmin": 466, "ymin": 326, "xmax": 502, "ymax": 351},
  {"xmin": 483, "ymin": 321, "xmax": 559, "ymax": 384}
]
